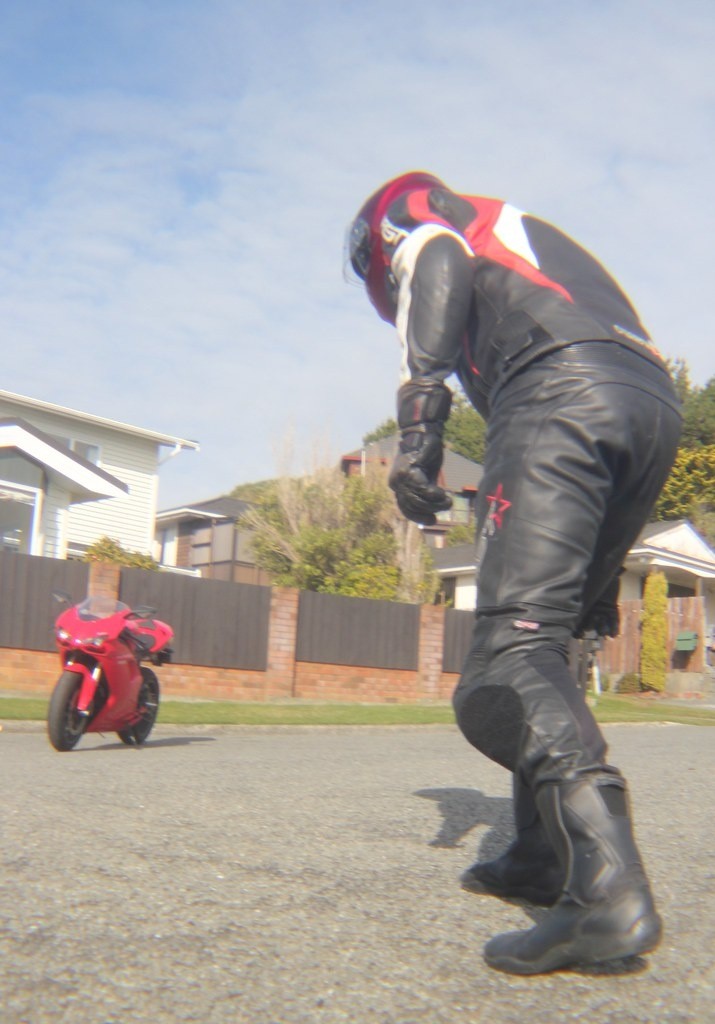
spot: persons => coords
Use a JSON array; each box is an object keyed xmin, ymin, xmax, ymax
[{"xmin": 340, "ymin": 172, "xmax": 687, "ymax": 975}]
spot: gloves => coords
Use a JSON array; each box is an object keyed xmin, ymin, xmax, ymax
[
  {"xmin": 389, "ymin": 379, "xmax": 457, "ymax": 527},
  {"xmin": 572, "ymin": 576, "xmax": 622, "ymax": 641}
]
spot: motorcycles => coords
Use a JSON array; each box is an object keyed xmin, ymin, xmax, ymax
[{"xmin": 47, "ymin": 591, "xmax": 173, "ymax": 751}]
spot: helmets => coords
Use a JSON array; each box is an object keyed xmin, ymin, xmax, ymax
[{"xmin": 341, "ymin": 172, "xmax": 449, "ymax": 328}]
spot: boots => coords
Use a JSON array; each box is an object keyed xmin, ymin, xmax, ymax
[
  {"xmin": 463, "ymin": 771, "xmax": 565, "ymax": 902},
  {"xmin": 483, "ymin": 765, "xmax": 665, "ymax": 975}
]
